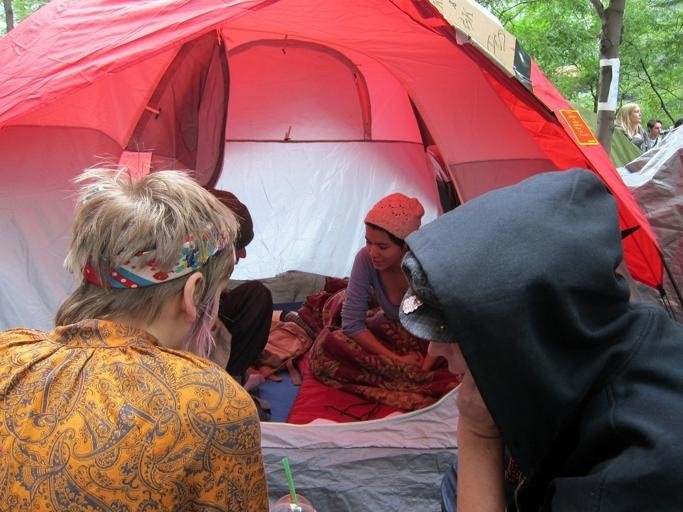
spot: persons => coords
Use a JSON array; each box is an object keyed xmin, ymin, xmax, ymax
[
  {"xmin": 279, "ymin": 192, "xmax": 443, "ymax": 366},
  {"xmin": 1, "ymin": 163, "xmax": 317, "ymax": 511},
  {"xmin": 400, "ymin": 170, "xmax": 682, "ymax": 510},
  {"xmin": 616, "ymin": 103, "xmax": 683, "ymax": 151}
]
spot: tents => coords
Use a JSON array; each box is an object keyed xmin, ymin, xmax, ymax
[{"xmin": 1, "ymin": 0, "xmax": 683, "ymax": 512}]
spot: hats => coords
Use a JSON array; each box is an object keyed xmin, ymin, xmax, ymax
[
  {"xmin": 364, "ymin": 193, "xmax": 425, "ymax": 239},
  {"xmin": 204, "ymin": 186, "xmax": 254, "ymax": 252},
  {"xmin": 397, "ymin": 249, "xmax": 457, "ymax": 345}
]
